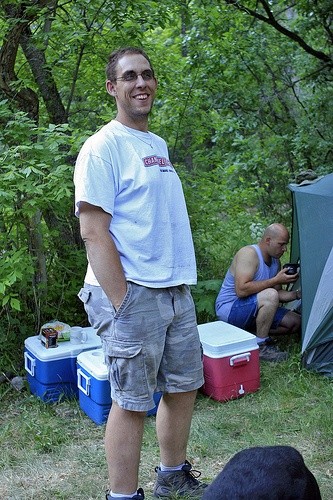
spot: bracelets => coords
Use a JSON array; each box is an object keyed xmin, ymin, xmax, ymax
[{"xmin": 295, "ymin": 290, "xmax": 300, "ymax": 300}]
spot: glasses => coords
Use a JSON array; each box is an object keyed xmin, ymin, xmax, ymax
[{"xmin": 113, "ymin": 70, "xmax": 154, "ymax": 83}]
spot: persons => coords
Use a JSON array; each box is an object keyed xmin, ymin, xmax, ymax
[
  {"xmin": 73, "ymin": 48, "xmax": 206, "ymax": 500},
  {"xmin": 215, "ymin": 223, "xmax": 302, "ymax": 361}
]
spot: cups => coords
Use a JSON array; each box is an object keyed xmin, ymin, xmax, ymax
[{"xmin": 70, "ymin": 326, "xmax": 88, "ymax": 345}]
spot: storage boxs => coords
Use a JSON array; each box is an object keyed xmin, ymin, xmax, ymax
[
  {"xmin": 76, "ymin": 349, "xmax": 162, "ymax": 425},
  {"xmin": 197, "ymin": 321, "xmax": 260, "ymax": 403},
  {"xmin": 23, "ymin": 327, "xmax": 104, "ymax": 402}
]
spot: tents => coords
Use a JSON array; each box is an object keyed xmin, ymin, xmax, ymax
[{"xmin": 279, "ymin": 172, "xmax": 333, "ymax": 378}]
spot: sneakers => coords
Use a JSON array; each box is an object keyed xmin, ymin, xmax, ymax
[
  {"xmin": 152, "ymin": 460, "xmax": 209, "ymax": 500},
  {"xmin": 258, "ymin": 337, "xmax": 293, "ymax": 363}
]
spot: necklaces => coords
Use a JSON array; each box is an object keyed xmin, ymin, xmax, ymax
[{"xmin": 123, "ymin": 125, "xmax": 154, "ymax": 149}]
